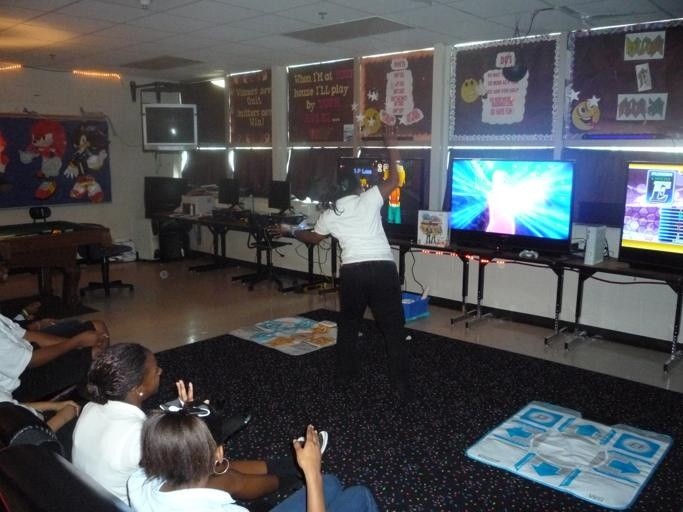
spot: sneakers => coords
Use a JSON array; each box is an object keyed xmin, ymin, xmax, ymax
[{"xmin": 317, "ymin": 431, "xmax": 328, "ymax": 456}]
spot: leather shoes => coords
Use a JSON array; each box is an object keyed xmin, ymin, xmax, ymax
[{"xmin": 214, "ymin": 409, "xmax": 253, "ymax": 443}]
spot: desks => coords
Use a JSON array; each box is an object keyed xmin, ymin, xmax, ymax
[
  {"xmin": 0, "ymin": 221, "xmax": 112, "ymax": 314},
  {"xmin": 153, "ymin": 212, "xmax": 682, "ymax": 371}
]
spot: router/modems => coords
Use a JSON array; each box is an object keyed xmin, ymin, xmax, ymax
[{"xmin": 583, "ymin": 225, "xmax": 604, "ymax": 265}]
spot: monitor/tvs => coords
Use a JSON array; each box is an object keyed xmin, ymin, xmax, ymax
[
  {"xmin": 618, "ymin": 160, "xmax": 683, "ymax": 274},
  {"xmin": 268, "ymin": 181, "xmax": 290, "ymax": 208},
  {"xmin": 219, "ymin": 179, "xmax": 238, "ymax": 204},
  {"xmin": 338, "ymin": 156, "xmax": 424, "ymax": 234},
  {"xmin": 142, "ymin": 104, "xmax": 197, "ymax": 150},
  {"xmin": 445, "ymin": 156, "xmax": 574, "ymax": 253}
]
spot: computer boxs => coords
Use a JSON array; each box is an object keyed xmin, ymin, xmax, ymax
[
  {"xmin": 212, "ymin": 210, "xmax": 250, "ymax": 222},
  {"xmin": 260, "ymin": 214, "xmax": 303, "ymax": 226}
]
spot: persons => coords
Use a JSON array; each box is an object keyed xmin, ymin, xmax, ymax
[
  {"xmin": 72, "ymin": 341, "xmax": 377, "ymax": 511},
  {"xmin": 1, "ymin": 299, "xmax": 108, "ymax": 433},
  {"xmin": 267, "ymin": 124, "xmax": 412, "ymax": 408}
]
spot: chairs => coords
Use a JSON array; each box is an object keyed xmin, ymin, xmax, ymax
[
  {"xmin": 76, "ymin": 225, "xmax": 135, "ymax": 299},
  {"xmin": 2, "ymin": 399, "xmax": 62, "ymax": 449},
  {"xmin": 28, "ymin": 205, "xmax": 50, "ymax": 222},
  {"xmin": 0, "ymin": 439, "xmax": 139, "ymax": 512}
]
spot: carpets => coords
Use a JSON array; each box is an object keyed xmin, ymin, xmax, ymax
[{"xmin": 81, "ymin": 307, "xmax": 682, "ymax": 512}]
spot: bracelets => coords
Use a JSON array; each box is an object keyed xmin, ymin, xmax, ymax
[{"xmin": 290, "ymin": 228, "xmax": 296, "ymax": 239}]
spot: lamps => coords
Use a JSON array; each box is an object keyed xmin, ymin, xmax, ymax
[{"xmin": 503, "ymin": 19, "xmax": 528, "ymax": 83}]
